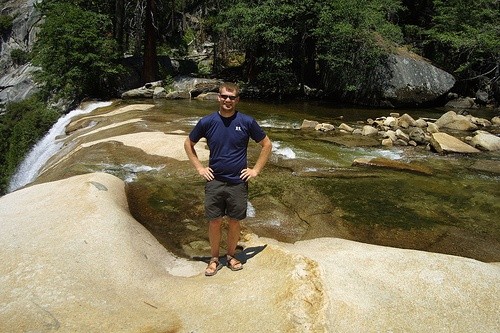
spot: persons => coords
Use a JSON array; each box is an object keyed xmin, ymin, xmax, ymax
[{"xmin": 184, "ymin": 82, "xmax": 272, "ymax": 276}]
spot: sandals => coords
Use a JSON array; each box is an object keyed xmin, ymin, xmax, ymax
[
  {"xmin": 226, "ymin": 257, "xmax": 243, "ymax": 272},
  {"xmin": 205, "ymin": 258, "xmax": 223, "ymax": 274}
]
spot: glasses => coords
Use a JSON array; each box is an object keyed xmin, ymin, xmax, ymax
[{"xmin": 220, "ymin": 95, "xmax": 239, "ymax": 100}]
